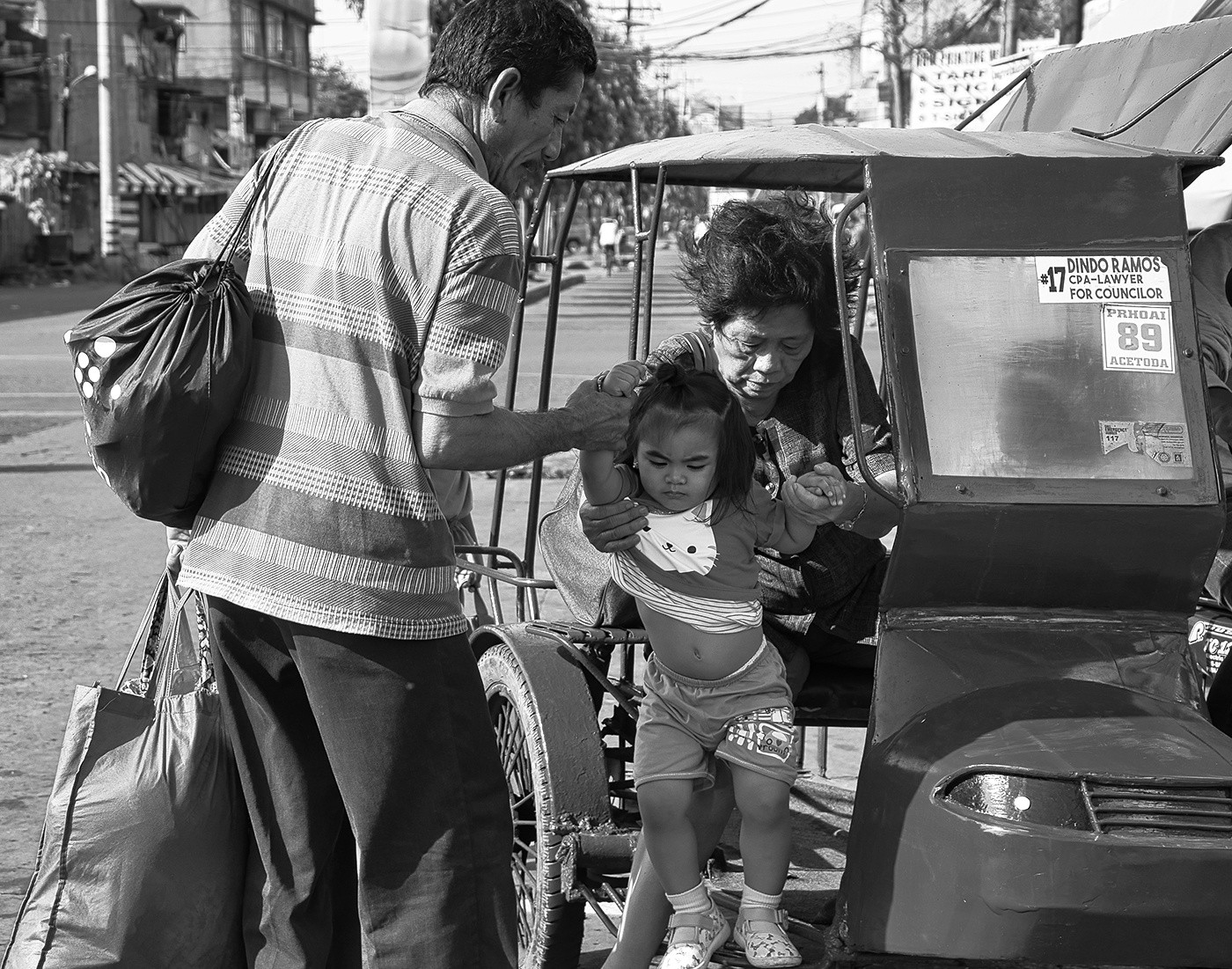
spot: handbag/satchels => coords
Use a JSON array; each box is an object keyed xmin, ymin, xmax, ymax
[
  {"xmin": 2, "ymin": 543, "xmax": 249, "ymax": 969},
  {"xmin": 537, "ymin": 332, "xmax": 715, "ymax": 628},
  {"xmin": 63, "ymin": 118, "xmax": 327, "ymax": 530}
]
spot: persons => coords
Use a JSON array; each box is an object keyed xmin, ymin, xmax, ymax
[
  {"xmin": 578, "ymin": 361, "xmax": 850, "ymax": 968},
  {"xmin": 580, "ymin": 187, "xmax": 906, "ymax": 967},
  {"xmin": 1184, "ymin": 219, "xmax": 1232, "ymax": 735},
  {"xmin": 166, "ymin": 0, "xmax": 637, "ymax": 969},
  {"xmin": 599, "ymin": 217, "xmax": 618, "ymax": 276},
  {"xmin": 695, "ymin": 214, "xmax": 712, "ymax": 245}
]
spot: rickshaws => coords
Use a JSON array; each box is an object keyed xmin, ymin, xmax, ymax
[
  {"xmin": 600, "ymin": 227, "xmax": 648, "ymax": 277},
  {"xmin": 447, "ymin": 0, "xmax": 1232, "ymax": 969}
]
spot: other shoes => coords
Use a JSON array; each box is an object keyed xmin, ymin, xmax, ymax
[
  {"xmin": 733, "ymin": 907, "xmax": 802, "ymax": 968},
  {"xmin": 657, "ymin": 887, "xmax": 730, "ymax": 969}
]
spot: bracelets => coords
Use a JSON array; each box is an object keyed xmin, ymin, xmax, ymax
[
  {"xmin": 838, "ymin": 482, "xmax": 868, "ymax": 530},
  {"xmin": 597, "ymin": 375, "xmax": 605, "ymax": 392}
]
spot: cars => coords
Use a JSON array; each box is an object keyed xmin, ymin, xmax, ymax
[{"xmin": 537, "ymin": 210, "xmax": 592, "ymax": 254}]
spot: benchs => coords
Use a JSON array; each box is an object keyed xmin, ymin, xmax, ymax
[{"xmin": 542, "ymin": 494, "xmax": 818, "ymax": 628}]
